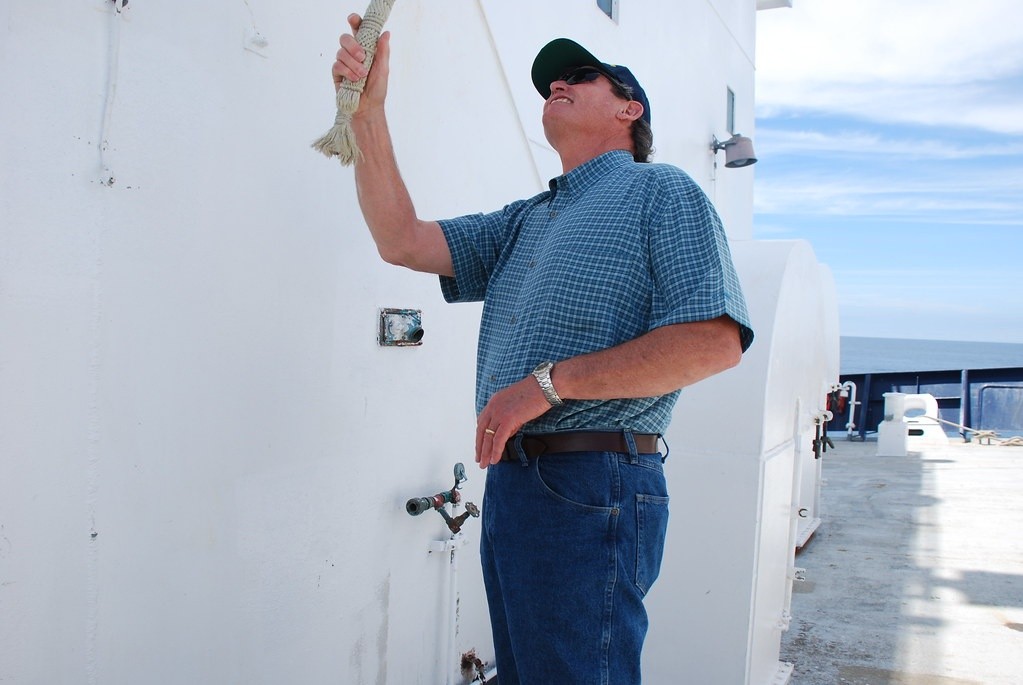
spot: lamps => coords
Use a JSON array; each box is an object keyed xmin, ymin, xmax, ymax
[{"xmin": 711, "ymin": 133, "xmax": 759, "ymax": 169}]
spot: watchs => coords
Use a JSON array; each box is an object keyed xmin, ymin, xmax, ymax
[{"xmin": 532, "ymin": 360, "xmax": 564, "ymax": 406}]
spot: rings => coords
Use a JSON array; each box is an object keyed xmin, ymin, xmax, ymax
[{"xmin": 484, "ymin": 429, "xmax": 495, "ymax": 436}]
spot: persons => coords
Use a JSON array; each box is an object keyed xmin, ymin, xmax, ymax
[{"xmin": 331, "ymin": 12, "xmax": 754, "ymax": 685}]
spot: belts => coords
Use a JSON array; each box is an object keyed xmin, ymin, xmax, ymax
[{"xmin": 499, "ymin": 433, "xmax": 659, "ymax": 461}]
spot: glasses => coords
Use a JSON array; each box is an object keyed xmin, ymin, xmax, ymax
[{"xmin": 558, "ymin": 67, "xmax": 631, "ymax": 102}]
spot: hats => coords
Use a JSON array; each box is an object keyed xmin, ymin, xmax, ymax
[{"xmin": 532, "ymin": 39, "xmax": 650, "ymax": 126}]
